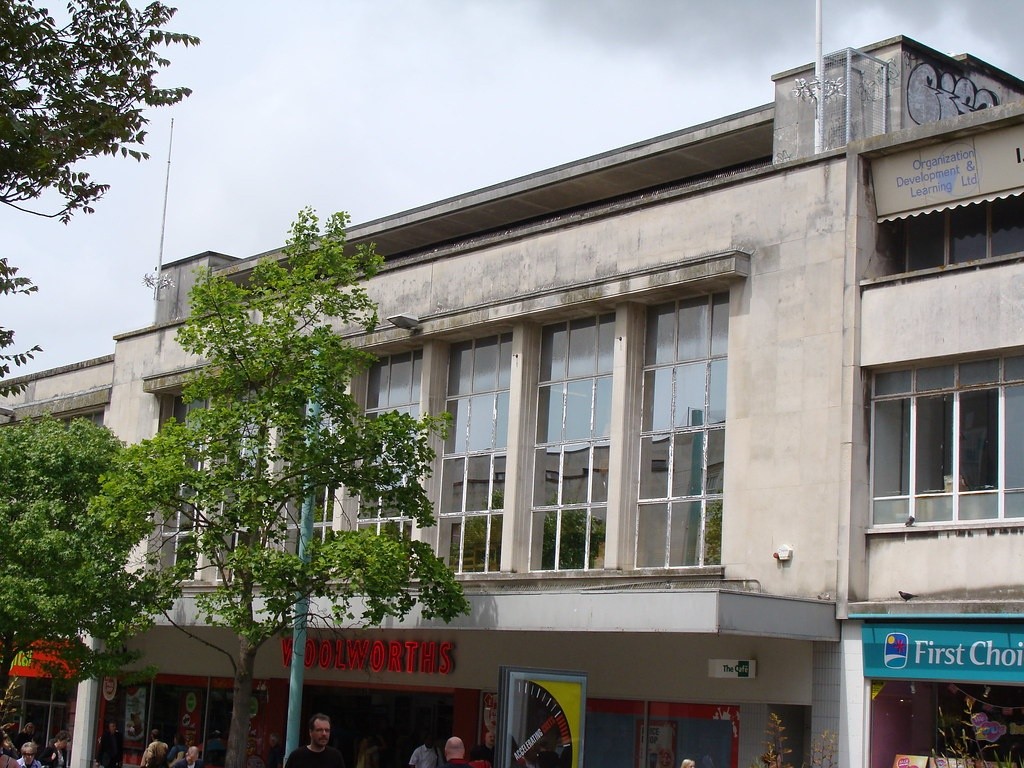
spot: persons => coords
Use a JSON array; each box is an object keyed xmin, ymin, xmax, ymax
[
  {"xmin": 16, "ymin": 742, "xmax": 42, "ymax": 768},
  {"xmin": 437, "ymin": 736, "xmax": 474, "ymax": 768},
  {"xmin": 408, "ymin": 732, "xmax": 438, "ymax": 768},
  {"xmin": 173, "ymin": 746, "xmax": 204, "ymax": 768},
  {"xmin": 352, "ymin": 726, "xmax": 400, "ymax": 768},
  {"xmin": 140, "ymin": 729, "xmax": 168, "ymax": 768},
  {"xmin": 468, "ymin": 731, "xmax": 495, "ymax": 762},
  {"xmin": 267, "ymin": 732, "xmax": 281, "ymax": 768},
  {"xmin": 96, "ymin": 719, "xmax": 123, "ymax": 768},
  {"xmin": 521, "ymin": 744, "xmax": 540, "ymax": 768},
  {"xmin": 284, "ymin": 713, "xmax": 345, "ymax": 768},
  {"xmin": 405, "ymin": 733, "xmax": 422, "ymax": 764},
  {"xmin": 0, "ymin": 730, "xmax": 21, "ymax": 768},
  {"xmin": 681, "ymin": 759, "xmax": 695, "ymax": 768},
  {"xmin": 13, "ymin": 722, "xmax": 35, "ymax": 750},
  {"xmin": 167, "ymin": 734, "xmax": 188, "ymax": 761},
  {"xmin": 536, "ymin": 740, "xmax": 560, "ymax": 768},
  {"xmin": 38, "ymin": 730, "xmax": 71, "ymax": 768},
  {"xmin": 559, "ymin": 736, "xmax": 572, "ymax": 768}
]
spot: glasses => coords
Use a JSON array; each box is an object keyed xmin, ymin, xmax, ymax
[
  {"xmin": 27, "ymin": 726, "xmax": 33, "ymax": 729},
  {"xmin": 24, "ymin": 753, "xmax": 35, "ymax": 756}
]
